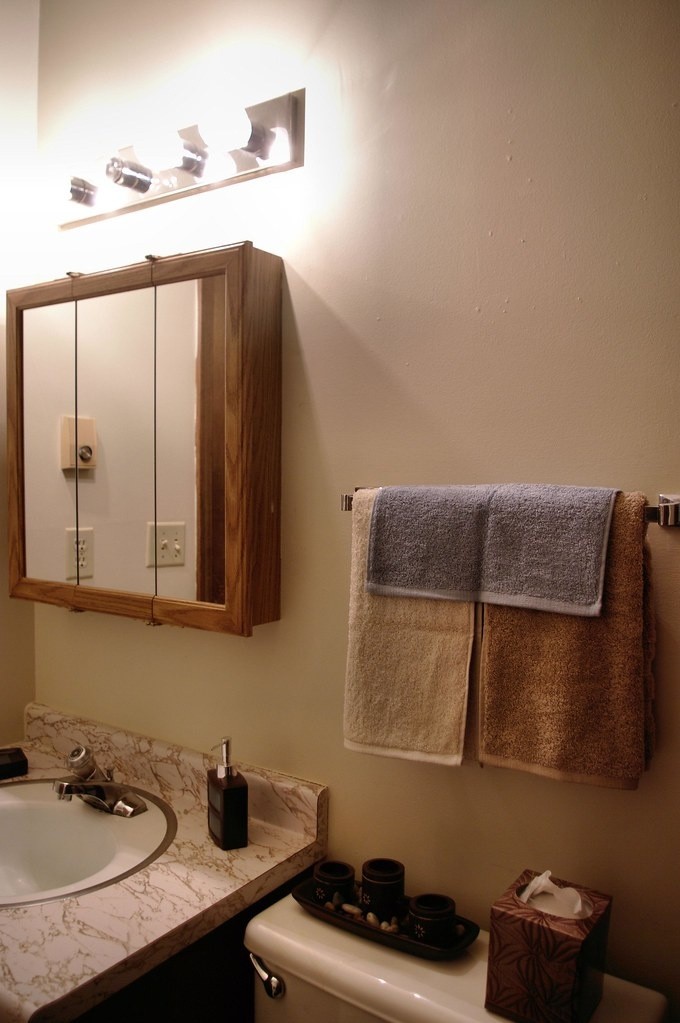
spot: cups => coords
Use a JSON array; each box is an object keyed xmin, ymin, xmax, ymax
[
  {"xmin": 311, "ymin": 862, "xmax": 356, "ymax": 905},
  {"xmin": 362, "ymin": 858, "xmax": 404, "ymax": 920},
  {"xmin": 408, "ymin": 894, "xmax": 456, "ymax": 944}
]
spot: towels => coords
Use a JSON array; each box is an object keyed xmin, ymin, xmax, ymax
[
  {"xmin": 474, "ymin": 492, "xmax": 658, "ymax": 792},
  {"xmin": 366, "ymin": 482, "xmax": 624, "ymax": 617},
  {"xmin": 342, "ymin": 486, "xmax": 479, "ymax": 770}
]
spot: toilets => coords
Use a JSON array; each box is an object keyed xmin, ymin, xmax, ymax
[{"xmin": 242, "ymin": 877, "xmax": 668, "ymax": 1023}]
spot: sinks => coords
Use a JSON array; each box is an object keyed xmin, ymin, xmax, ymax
[{"xmin": 0, "ymin": 777, "xmax": 178, "ymax": 908}]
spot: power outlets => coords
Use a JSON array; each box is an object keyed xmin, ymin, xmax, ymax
[{"xmin": 63, "ymin": 527, "xmax": 96, "ymax": 579}]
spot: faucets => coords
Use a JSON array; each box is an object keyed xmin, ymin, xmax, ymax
[{"xmin": 52, "ymin": 745, "xmax": 148, "ymax": 819}]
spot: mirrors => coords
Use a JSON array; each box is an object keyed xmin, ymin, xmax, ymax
[{"xmin": 5, "ymin": 239, "xmax": 282, "ymax": 640}]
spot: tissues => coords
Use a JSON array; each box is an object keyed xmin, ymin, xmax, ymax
[{"xmin": 484, "ymin": 868, "xmax": 614, "ymax": 1023}]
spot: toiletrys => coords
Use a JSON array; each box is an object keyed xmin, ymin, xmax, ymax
[{"xmin": 206, "ymin": 737, "xmax": 249, "ymax": 851}]
